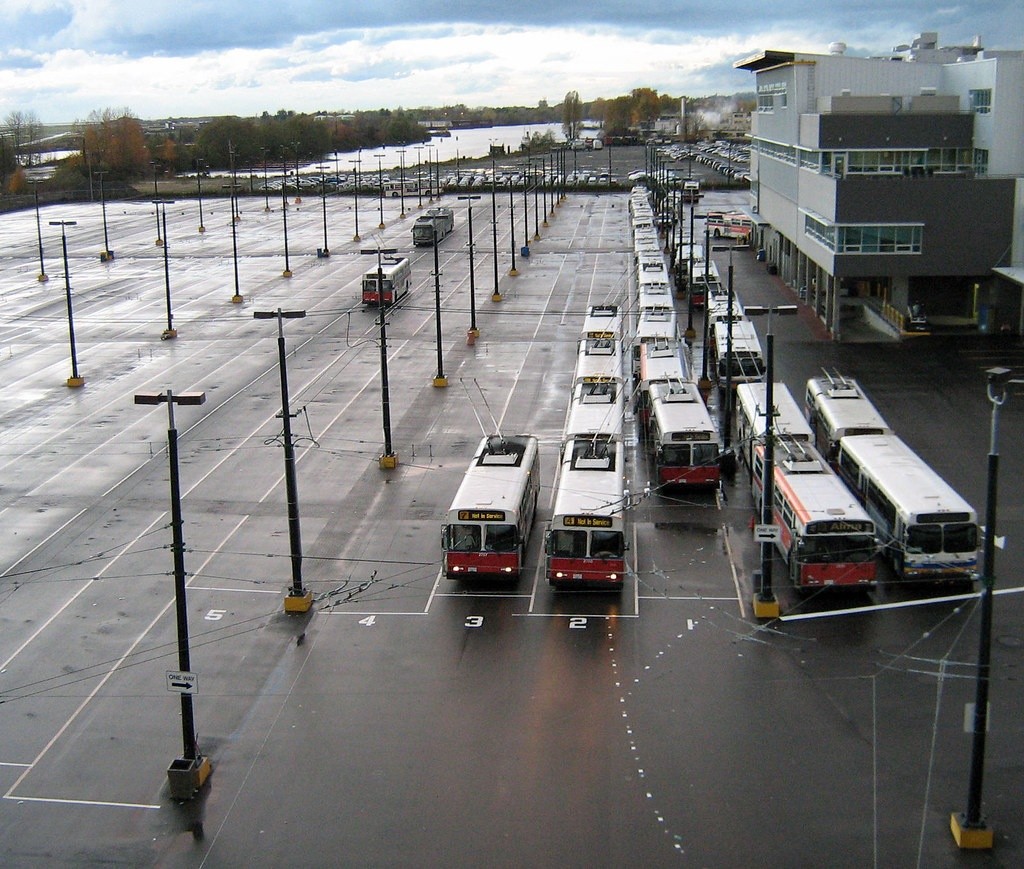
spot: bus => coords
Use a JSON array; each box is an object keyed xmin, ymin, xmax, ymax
[
  {"xmin": 410, "ymin": 206, "xmax": 455, "ymax": 247},
  {"xmin": 703, "ymin": 210, "xmax": 753, "ymax": 239},
  {"xmin": 439, "ymin": 186, "xmax": 720, "ymax": 590},
  {"xmin": 360, "ymin": 257, "xmax": 412, "ymax": 306},
  {"xmin": 382, "ymin": 179, "xmax": 443, "ymax": 197},
  {"xmin": 668, "ymin": 232, "xmax": 980, "ymax": 595}
]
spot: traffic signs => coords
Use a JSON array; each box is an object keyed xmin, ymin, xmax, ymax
[
  {"xmin": 165, "ymin": 670, "xmax": 198, "ymax": 694},
  {"xmin": 755, "ymin": 524, "xmax": 781, "ymax": 543}
]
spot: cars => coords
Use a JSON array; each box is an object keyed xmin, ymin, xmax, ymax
[
  {"xmin": 258, "ymin": 140, "xmax": 619, "ymax": 187},
  {"xmin": 628, "ymin": 139, "xmax": 751, "ymax": 231}
]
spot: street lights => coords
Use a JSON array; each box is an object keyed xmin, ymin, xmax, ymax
[
  {"xmin": 650, "ymin": 147, "xmax": 705, "ymax": 338},
  {"xmin": 420, "ymin": 215, "xmax": 450, "ymax": 387},
  {"xmin": 950, "ymin": 367, "xmax": 1024, "ymax": 849},
  {"xmin": 134, "ymin": 389, "xmax": 211, "ymax": 786},
  {"xmin": 360, "ymin": 247, "xmax": 400, "ymax": 469},
  {"xmin": 254, "ymin": 309, "xmax": 313, "ymax": 612},
  {"xmin": 743, "ymin": 304, "xmax": 798, "ymax": 617},
  {"xmin": 693, "ymin": 212, "xmax": 723, "ymax": 390},
  {"xmin": 713, "ymin": 245, "xmax": 751, "ymax": 468},
  {"xmin": 26, "ymin": 145, "xmax": 569, "ymax": 387}
]
